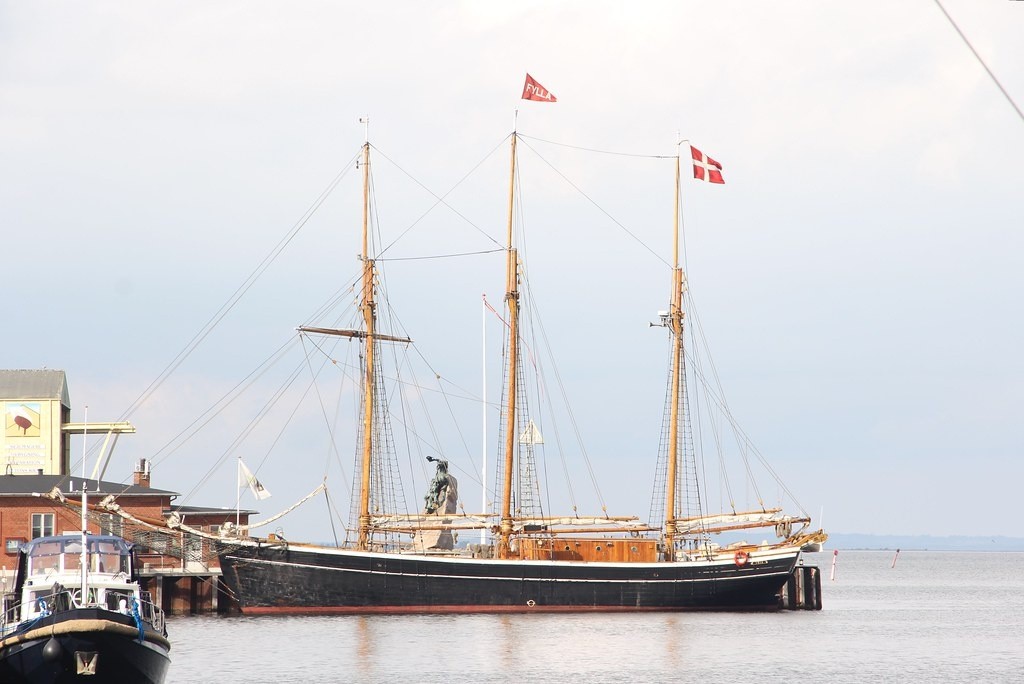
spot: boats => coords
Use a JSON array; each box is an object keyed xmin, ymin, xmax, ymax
[{"xmin": 0, "ymin": 481, "xmax": 173, "ymax": 684}]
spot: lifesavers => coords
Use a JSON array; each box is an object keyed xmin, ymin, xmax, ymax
[{"xmin": 733, "ymin": 551, "xmax": 749, "ymax": 567}]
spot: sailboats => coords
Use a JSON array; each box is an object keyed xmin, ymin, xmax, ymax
[{"xmin": 33, "ymin": 108, "xmax": 829, "ymax": 614}]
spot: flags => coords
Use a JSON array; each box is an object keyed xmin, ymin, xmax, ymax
[
  {"xmin": 690, "ymin": 145, "xmax": 725, "ymax": 184},
  {"xmin": 521, "ymin": 73, "xmax": 557, "ymax": 102},
  {"xmin": 237, "ymin": 457, "xmax": 271, "ymax": 501}
]
